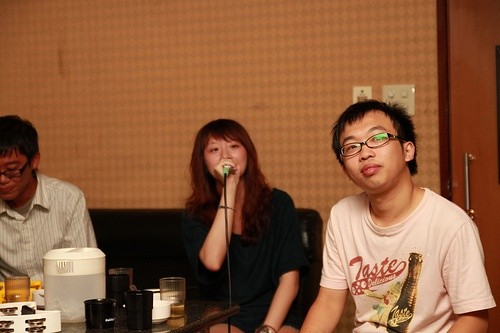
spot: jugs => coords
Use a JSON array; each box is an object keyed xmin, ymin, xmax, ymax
[{"xmin": 41, "ymin": 247, "xmax": 106, "ymax": 323}]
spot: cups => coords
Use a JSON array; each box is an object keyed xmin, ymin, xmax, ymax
[
  {"xmin": 4, "ymin": 276, "xmax": 30, "ymax": 304},
  {"xmin": 32, "ymin": 289, "xmax": 45, "ymax": 307},
  {"xmin": 83, "ymin": 268, "xmax": 185, "ymax": 333}
]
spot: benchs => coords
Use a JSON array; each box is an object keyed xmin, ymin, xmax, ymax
[{"xmin": 86, "ymin": 208, "xmax": 323, "ymax": 325}]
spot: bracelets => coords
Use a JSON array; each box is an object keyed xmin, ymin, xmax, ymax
[
  {"xmin": 218, "ymin": 206, "xmax": 236, "ymax": 211},
  {"xmin": 255, "ymin": 324, "xmax": 277, "ymax": 333}
]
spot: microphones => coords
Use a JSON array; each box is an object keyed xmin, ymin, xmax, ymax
[{"xmin": 223, "ymin": 165, "xmax": 231, "ymax": 175}]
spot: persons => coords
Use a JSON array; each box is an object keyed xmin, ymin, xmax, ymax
[
  {"xmin": 299, "ymin": 100, "xmax": 496, "ymax": 333},
  {"xmin": 0, "ymin": 115, "xmax": 98, "ymax": 286},
  {"xmin": 178, "ymin": 119, "xmax": 302, "ymax": 333}
]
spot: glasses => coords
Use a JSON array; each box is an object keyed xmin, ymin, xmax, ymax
[
  {"xmin": 340, "ymin": 132, "xmax": 407, "ymax": 160},
  {"xmin": 0, "ymin": 160, "xmax": 29, "ymax": 178}
]
